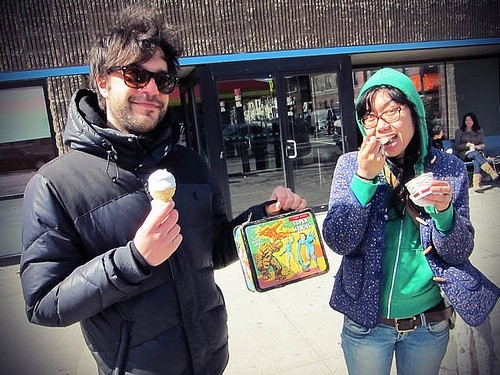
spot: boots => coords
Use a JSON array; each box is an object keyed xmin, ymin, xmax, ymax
[
  {"xmin": 480, "ymin": 162, "xmax": 498, "ymax": 180},
  {"xmin": 473, "ymin": 174, "xmax": 484, "ymax": 193}
]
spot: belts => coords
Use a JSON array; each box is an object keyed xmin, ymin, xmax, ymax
[{"xmin": 377, "ymin": 299, "xmax": 454, "ymax": 332}]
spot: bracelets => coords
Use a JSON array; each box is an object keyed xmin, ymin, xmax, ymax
[{"xmin": 354, "ymin": 172, "xmax": 375, "ymax": 181}]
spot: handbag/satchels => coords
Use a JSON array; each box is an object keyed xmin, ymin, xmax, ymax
[{"xmin": 233, "ymin": 207, "xmax": 330, "ymax": 292}]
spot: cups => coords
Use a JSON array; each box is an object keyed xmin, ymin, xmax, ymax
[{"xmin": 404, "ymin": 172, "xmax": 437, "ymax": 200}]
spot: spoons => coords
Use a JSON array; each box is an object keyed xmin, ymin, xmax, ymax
[{"xmin": 375, "ymin": 138, "xmax": 389, "ymax": 145}]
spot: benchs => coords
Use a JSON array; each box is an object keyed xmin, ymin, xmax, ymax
[{"xmin": 441, "ymin": 134, "xmax": 500, "ymax": 183}]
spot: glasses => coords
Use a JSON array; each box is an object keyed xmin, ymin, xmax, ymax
[
  {"xmin": 359, "ymin": 105, "xmax": 406, "ymax": 129},
  {"xmin": 110, "ymin": 65, "xmax": 179, "ymax": 94}
]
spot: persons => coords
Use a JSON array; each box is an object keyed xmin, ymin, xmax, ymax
[
  {"xmin": 23, "ymin": 9, "xmax": 307, "ymax": 375},
  {"xmin": 322, "ymin": 67, "xmax": 500, "ymax": 375}
]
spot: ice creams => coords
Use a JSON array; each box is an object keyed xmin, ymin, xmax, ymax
[{"xmin": 148, "ymin": 169, "xmax": 177, "ymax": 201}]
